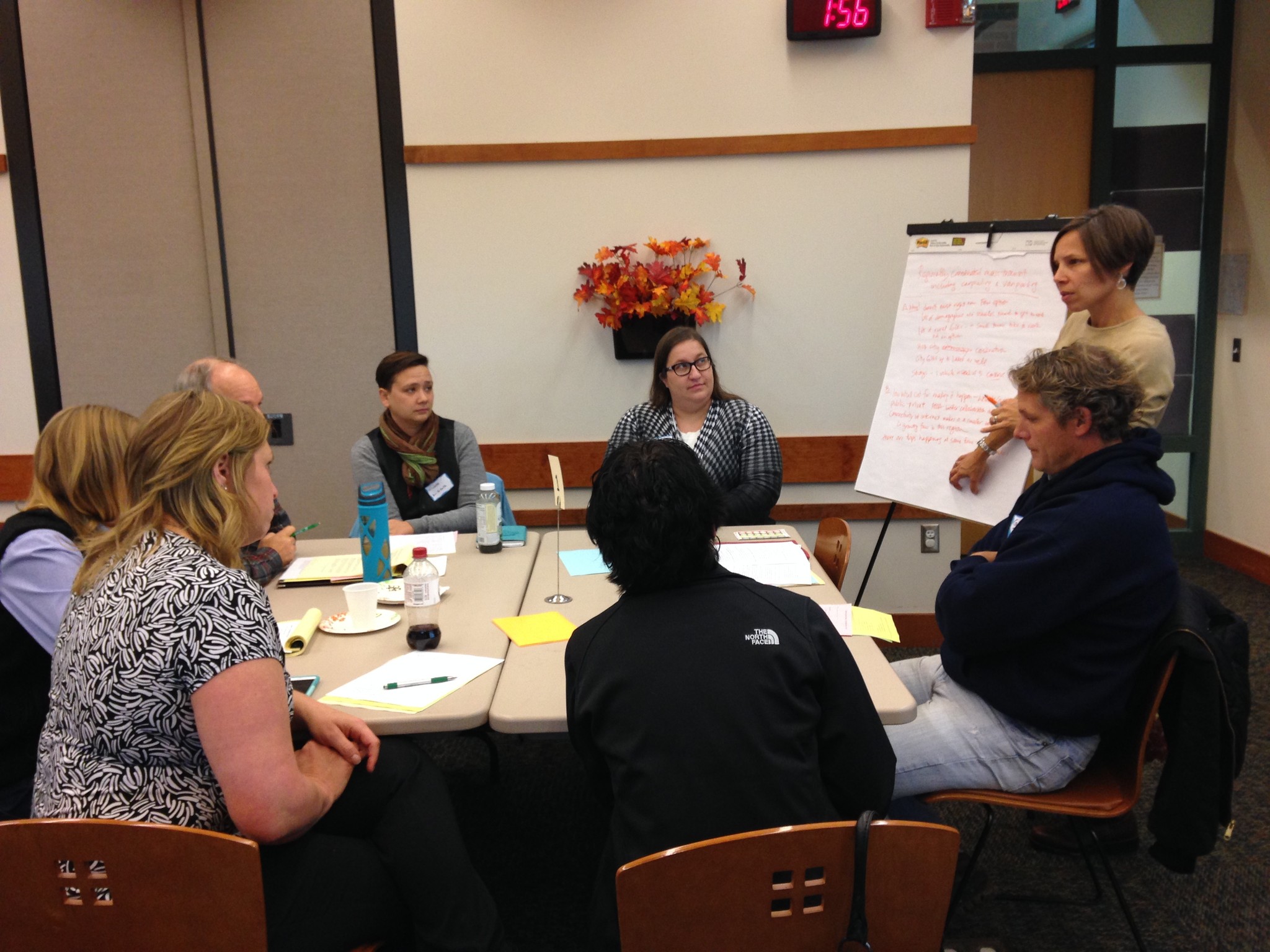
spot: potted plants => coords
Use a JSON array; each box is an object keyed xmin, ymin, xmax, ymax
[{"xmin": 572, "ymin": 237, "xmax": 757, "ymax": 363}]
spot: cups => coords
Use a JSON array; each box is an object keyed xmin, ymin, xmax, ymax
[{"xmin": 343, "ymin": 582, "xmax": 379, "ymax": 622}]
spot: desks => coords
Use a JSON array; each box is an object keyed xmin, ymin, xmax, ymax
[
  {"xmin": 488, "ymin": 524, "xmax": 921, "ymax": 805},
  {"xmin": 256, "ymin": 527, "xmax": 545, "ymax": 798}
]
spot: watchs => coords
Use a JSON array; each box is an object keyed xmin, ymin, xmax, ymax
[{"xmin": 977, "ymin": 437, "xmax": 997, "ymax": 456}]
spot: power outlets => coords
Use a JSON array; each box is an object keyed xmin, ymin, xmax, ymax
[{"xmin": 920, "ymin": 524, "xmax": 940, "ymax": 554}]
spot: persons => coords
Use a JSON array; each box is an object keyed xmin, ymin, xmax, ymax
[
  {"xmin": 28, "ymin": 388, "xmax": 513, "ymax": 950},
  {"xmin": 0, "ymin": 406, "xmax": 139, "ymax": 820},
  {"xmin": 564, "ymin": 439, "xmax": 895, "ymax": 949},
  {"xmin": 949, "ymin": 203, "xmax": 1176, "ymax": 496},
  {"xmin": 173, "ymin": 358, "xmax": 296, "ymax": 585},
  {"xmin": 882, "ymin": 342, "xmax": 1175, "ymax": 797},
  {"xmin": 604, "ymin": 327, "xmax": 784, "ymax": 524},
  {"xmin": 349, "ymin": 351, "xmax": 487, "ymax": 536}
]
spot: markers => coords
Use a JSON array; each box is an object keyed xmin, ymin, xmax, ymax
[{"xmin": 983, "ymin": 393, "xmax": 1003, "ymax": 409}]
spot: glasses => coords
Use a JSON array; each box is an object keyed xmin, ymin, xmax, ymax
[{"xmin": 665, "ymin": 356, "xmax": 711, "ymax": 377}]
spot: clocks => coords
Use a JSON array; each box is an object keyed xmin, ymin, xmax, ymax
[{"xmin": 784, "ymin": 0, "xmax": 883, "ymax": 42}]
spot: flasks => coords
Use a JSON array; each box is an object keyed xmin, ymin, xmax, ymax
[{"xmin": 358, "ymin": 482, "xmax": 393, "ymax": 582}]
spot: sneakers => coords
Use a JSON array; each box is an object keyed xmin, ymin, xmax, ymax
[{"xmin": 1031, "ymin": 816, "xmax": 1138, "ymax": 854}]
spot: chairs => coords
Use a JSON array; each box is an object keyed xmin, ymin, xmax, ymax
[
  {"xmin": 613, "ymin": 819, "xmax": 961, "ymax": 952},
  {"xmin": 0, "ymin": 817, "xmax": 271, "ymax": 952},
  {"xmin": 920, "ymin": 647, "xmax": 1180, "ymax": 952},
  {"xmin": 813, "ymin": 518, "xmax": 852, "ymax": 591}
]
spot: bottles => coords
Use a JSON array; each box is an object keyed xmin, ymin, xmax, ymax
[
  {"xmin": 476, "ymin": 482, "xmax": 502, "ymax": 553},
  {"xmin": 404, "ymin": 547, "xmax": 443, "ymax": 652}
]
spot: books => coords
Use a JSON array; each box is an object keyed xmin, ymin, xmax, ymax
[
  {"xmin": 277, "ymin": 546, "xmax": 415, "ymax": 588},
  {"xmin": 275, "ymin": 607, "xmax": 322, "ymax": 656}
]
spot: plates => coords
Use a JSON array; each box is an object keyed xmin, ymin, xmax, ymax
[
  {"xmin": 318, "ymin": 609, "xmax": 401, "ymax": 634},
  {"xmin": 376, "ymin": 578, "xmax": 450, "ymax": 606}
]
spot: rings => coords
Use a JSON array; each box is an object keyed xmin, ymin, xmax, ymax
[{"xmin": 993, "ymin": 415, "xmax": 998, "ymax": 424}]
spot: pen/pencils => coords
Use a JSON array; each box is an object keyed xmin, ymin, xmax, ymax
[
  {"xmin": 383, "ymin": 676, "xmax": 458, "ymax": 690},
  {"xmin": 289, "ymin": 522, "xmax": 321, "ymax": 537}
]
